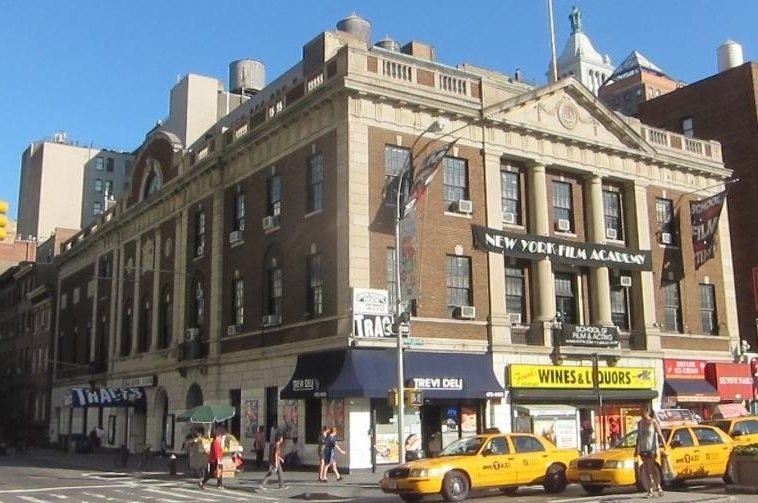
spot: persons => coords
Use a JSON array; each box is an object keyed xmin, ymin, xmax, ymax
[
  {"xmin": 582, "ymin": 420, "xmax": 596, "ymax": 455},
  {"xmin": 94, "ymin": 423, "xmax": 105, "ymax": 456},
  {"xmin": 197, "ymin": 432, "xmax": 225, "ymax": 491},
  {"xmin": 89, "ymin": 425, "xmax": 98, "ymax": 456},
  {"xmin": 259, "ymin": 433, "xmax": 290, "ymax": 491},
  {"xmin": 607, "ymin": 416, "xmax": 620, "ymax": 446},
  {"xmin": 634, "ymin": 405, "xmax": 668, "ymax": 498},
  {"xmin": 320, "ymin": 426, "xmax": 346, "ymax": 483},
  {"xmin": 404, "ymin": 432, "xmax": 421, "ymax": 451},
  {"xmin": 318, "ymin": 424, "xmax": 330, "ymax": 481},
  {"xmin": 255, "ymin": 426, "xmax": 266, "ymax": 469},
  {"xmin": 179, "ymin": 427, "xmax": 197, "ymax": 466}
]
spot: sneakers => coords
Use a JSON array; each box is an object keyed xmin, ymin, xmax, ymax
[
  {"xmin": 197, "ymin": 480, "xmax": 227, "ymax": 491},
  {"xmin": 647, "ymin": 486, "xmax": 665, "ymax": 499}
]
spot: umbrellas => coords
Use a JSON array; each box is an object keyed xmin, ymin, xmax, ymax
[{"xmin": 175, "ymin": 401, "xmax": 237, "ymax": 473}]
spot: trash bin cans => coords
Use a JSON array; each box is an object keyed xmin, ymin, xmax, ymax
[{"xmin": 406, "ymin": 450, "xmax": 425, "ymax": 461}]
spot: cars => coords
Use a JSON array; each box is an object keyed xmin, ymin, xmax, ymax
[
  {"xmin": 379, "ymin": 432, "xmax": 583, "ymax": 503},
  {"xmin": 564, "ymin": 423, "xmax": 739, "ymax": 494},
  {"xmin": 697, "ymin": 415, "xmax": 758, "ymax": 446}
]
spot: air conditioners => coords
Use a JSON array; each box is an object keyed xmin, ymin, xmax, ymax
[
  {"xmin": 457, "ymin": 305, "xmax": 522, "ymax": 324},
  {"xmin": 228, "ymin": 216, "xmax": 280, "ymax": 244},
  {"xmin": 454, "ymin": 198, "xmax": 673, "ymax": 246},
  {"xmin": 614, "ymin": 275, "xmax": 632, "ymax": 288},
  {"xmin": 227, "ymin": 314, "xmax": 278, "ymax": 336},
  {"xmin": 197, "ymin": 246, "xmax": 205, "ymax": 256},
  {"xmin": 186, "ymin": 328, "xmax": 200, "ymax": 342}
]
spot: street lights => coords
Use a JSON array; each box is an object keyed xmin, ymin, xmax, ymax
[{"xmin": 393, "ymin": 120, "xmax": 445, "ymax": 464}]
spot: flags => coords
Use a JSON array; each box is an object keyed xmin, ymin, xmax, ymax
[
  {"xmin": 687, "ymin": 186, "xmax": 728, "ymax": 270},
  {"xmin": 399, "ymin": 134, "xmax": 461, "ymax": 218}
]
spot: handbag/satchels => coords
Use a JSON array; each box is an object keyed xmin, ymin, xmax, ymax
[{"xmin": 213, "ymin": 465, "xmax": 223, "ymax": 479}]
